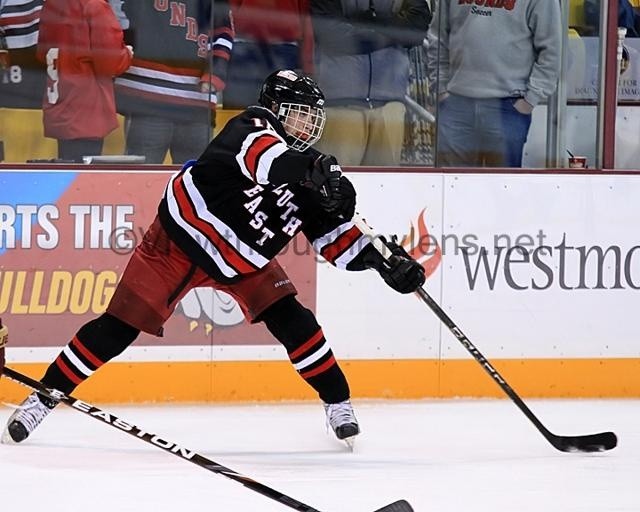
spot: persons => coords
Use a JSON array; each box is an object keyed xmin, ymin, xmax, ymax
[
  {"xmin": 298, "ymin": 1, "xmax": 434, "ymax": 167},
  {"xmin": 6, "ymin": 64, "xmax": 423, "ymax": 442},
  {"xmin": 423, "ymin": 0, "xmax": 563, "ymax": 169},
  {"xmin": 107, "ymin": 0, "xmax": 234, "ymax": 168},
  {"xmin": 32, "ymin": 0, "xmax": 135, "ymax": 164},
  {"xmin": 0, "ymin": 0, "xmax": 50, "ymax": 110},
  {"xmin": 579, "ymin": 0, "xmax": 640, "ymax": 42},
  {"xmin": 219, "ymin": 0, "xmax": 318, "ymax": 114}
]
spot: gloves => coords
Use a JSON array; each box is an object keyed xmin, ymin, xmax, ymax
[
  {"xmin": 300, "ymin": 153, "xmax": 357, "ymax": 222},
  {"xmin": 365, "ymin": 234, "xmax": 426, "ymax": 294}
]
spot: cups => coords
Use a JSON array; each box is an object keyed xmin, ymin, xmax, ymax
[{"xmin": 567, "ymin": 156, "xmax": 587, "ymax": 168}]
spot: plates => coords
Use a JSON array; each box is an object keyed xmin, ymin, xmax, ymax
[{"xmin": 83, "ymin": 155, "xmax": 147, "ymax": 165}]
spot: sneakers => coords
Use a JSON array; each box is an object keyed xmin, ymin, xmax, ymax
[
  {"xmin": 323, "ymin": 399, "xmax": 358, "ymax": 439},
  {"xmin": 7, "ymin": 391, "xmax": 52, "ymax": 443}
]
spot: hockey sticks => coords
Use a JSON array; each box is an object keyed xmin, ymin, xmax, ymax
[
  {"xmin": 1, "ymin": 365, "xmax": 413, "ymax": 512},
  {"xmin": 315, "ymin": 153, "xmax": 618, "ymax": 452}
]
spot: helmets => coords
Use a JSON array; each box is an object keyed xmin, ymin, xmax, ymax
[{"xmin": 257, "ymin": 67, "xmax": 327, "ymax": 153}]
